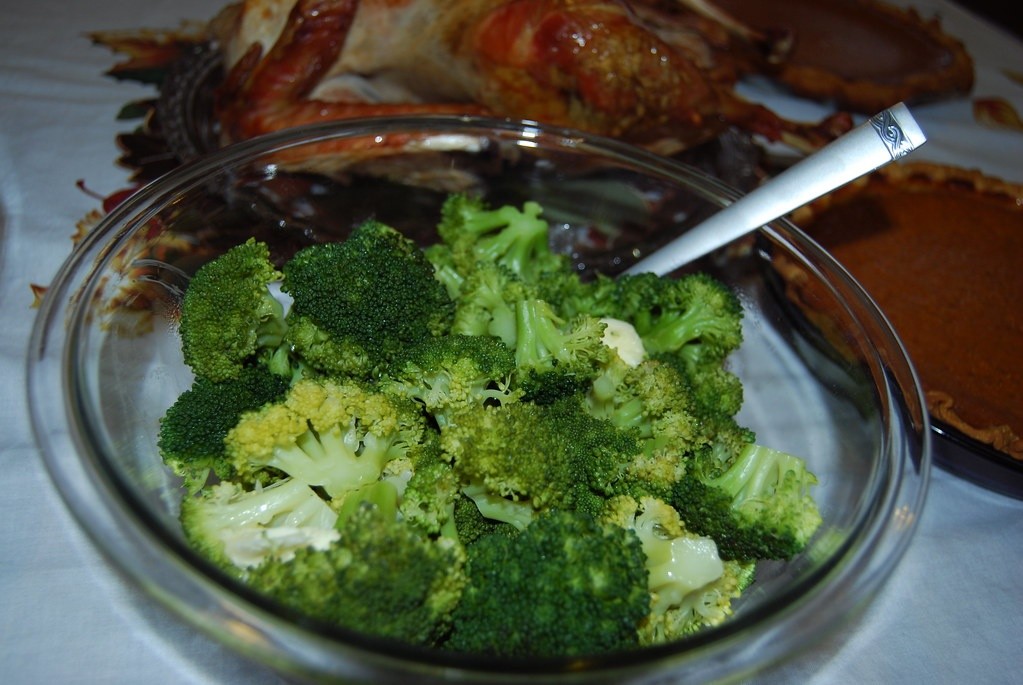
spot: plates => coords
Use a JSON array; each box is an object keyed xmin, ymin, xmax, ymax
[{"xmin": 26, "ymin": 115, "xmax": 931, "ymax": 685}]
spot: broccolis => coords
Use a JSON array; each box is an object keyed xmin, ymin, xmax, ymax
[{"xmin": 155, "ymin": 188, "xmax": 823, "ymax": 652}]
